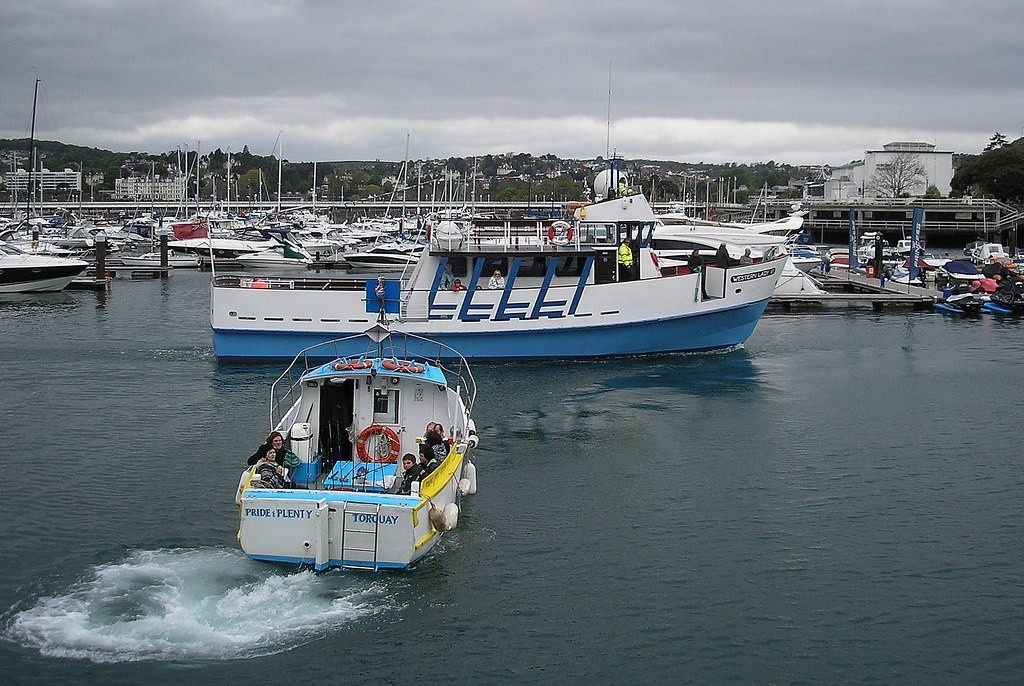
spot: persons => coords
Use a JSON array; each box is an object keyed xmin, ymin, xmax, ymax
[
  {"xmin": 618, "ymin": 238, "xmax": 635, "ymax": 281},
  {"xmin": 440, "ymin": 264, "xmax": 504, "ymax": 291},
  {"xmin": 687, "ymin": 243, "xmax": 753, "ymax": 300},
  {"xmin": 248, "ymin": 432, "xmax": 307, "ymax": 489},
  {"xmin": 395, "ymin": 421, "xmax": 450, "ymax": 494}
]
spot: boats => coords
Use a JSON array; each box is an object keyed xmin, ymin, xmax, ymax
[
  {"xmin": 0, "ymin": 76, "xmax": 1024, "ymax": 366},
  {"xmin": 232, "ymin": 296, "xmax": 477, "ymax": 571}
]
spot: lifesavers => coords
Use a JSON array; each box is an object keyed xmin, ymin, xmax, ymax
[
  {"xmin": 382, "ymin": 359, "xmax": 424, "ymax": 373},
  {"xmin": 332, "ymin": 359, "xmax": 372, "ymax": 370},
  {"xmin": 549, "ymin": 220, "xmax": 573, "ymax": 245},
  {"xmin": 357, "ymin": 425, "xmax": 400, "ymax": 464}
]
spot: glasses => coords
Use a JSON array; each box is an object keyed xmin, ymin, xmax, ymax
[
  {"xmin": 454, "ymin": 281, "xmax": 460, "ymax": 284},
  {"xmin": 625, "ymin": 241, "xmax": 630, "ymax": 243}
]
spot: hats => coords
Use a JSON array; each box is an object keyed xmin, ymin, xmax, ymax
[{"xmin": 259, "ymin": 445, "xmax": 274, "ymax": 458}]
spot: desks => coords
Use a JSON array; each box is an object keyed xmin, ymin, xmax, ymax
[{"xmin": 324, "ymin": 460, "xmax": 398, "ymax": 494}]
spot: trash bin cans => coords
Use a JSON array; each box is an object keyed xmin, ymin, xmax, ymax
[
  {"xmin": 290, "ymin": 423, "xmax": 314, "ymax": 464},
  {"xmin": 866, "ymin": 265, "xmax": 874, "ymax": 279},
  {"xmin": 943, "ymin": 289, "xmax": 952, "ymax": 298}
]
